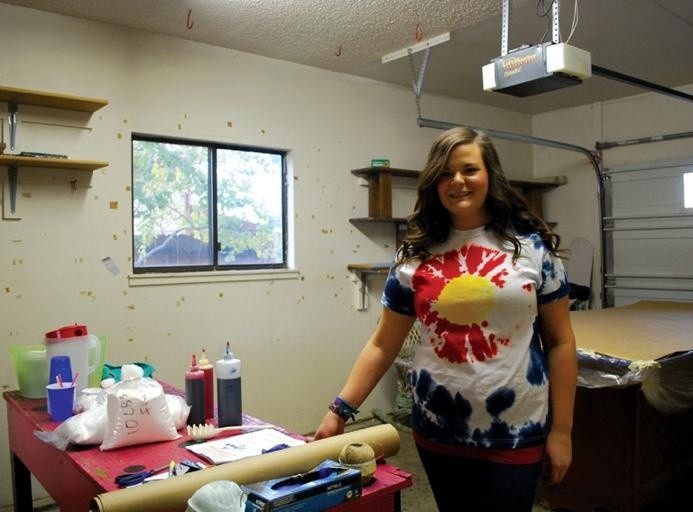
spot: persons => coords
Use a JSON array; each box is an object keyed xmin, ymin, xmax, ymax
[{"xmin": 304, "ymin": 127, "xmax": 579, "ymax": 511}]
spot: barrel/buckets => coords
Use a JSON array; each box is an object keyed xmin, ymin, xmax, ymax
[{"xmin": 45, "ymin": 322, "xmax": 102, "ymax": 414}]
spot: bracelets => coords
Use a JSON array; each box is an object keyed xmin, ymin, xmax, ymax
[{"xmin": 328, "ymin": 396, "xmax": 360, "ymax": 423}]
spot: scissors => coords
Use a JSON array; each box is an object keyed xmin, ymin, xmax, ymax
[{"xmin": 116, "ymin": 462, "xmax": 170, "ymax": 486}]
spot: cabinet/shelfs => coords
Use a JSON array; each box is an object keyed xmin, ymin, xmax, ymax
[
  {"xmin": 544, "ymin": 299, "xmax": 693, "ymax": 511},
  {"xmin": 348, "ymin": 165, "xmax": 560, "ymax": 271},
  {"xmin": 0, "ymin": 86, "xmax": 110, "ymax": 170}
]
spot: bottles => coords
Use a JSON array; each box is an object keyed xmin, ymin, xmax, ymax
[{"xmin": 186, "ymin": 339, "xmax": 243, "ymax": 430}]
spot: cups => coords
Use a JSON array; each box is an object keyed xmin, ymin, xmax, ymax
[
  {"xmin": 46, "ymin": 380, "xmax": 76, "ymax": 422},
  {"xmin": 10, "ymin": 323, "xmax": 107, "ymax": 403}
]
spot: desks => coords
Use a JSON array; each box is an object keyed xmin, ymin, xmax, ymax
[{"xmin": 4, "ymin": 377, "xmax": 413, "ymax": 512}]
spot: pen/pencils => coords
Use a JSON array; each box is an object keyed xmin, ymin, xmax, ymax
[{"xmin": 55, "ymin": 373, "xmax": 81, "ymax": 388}]
